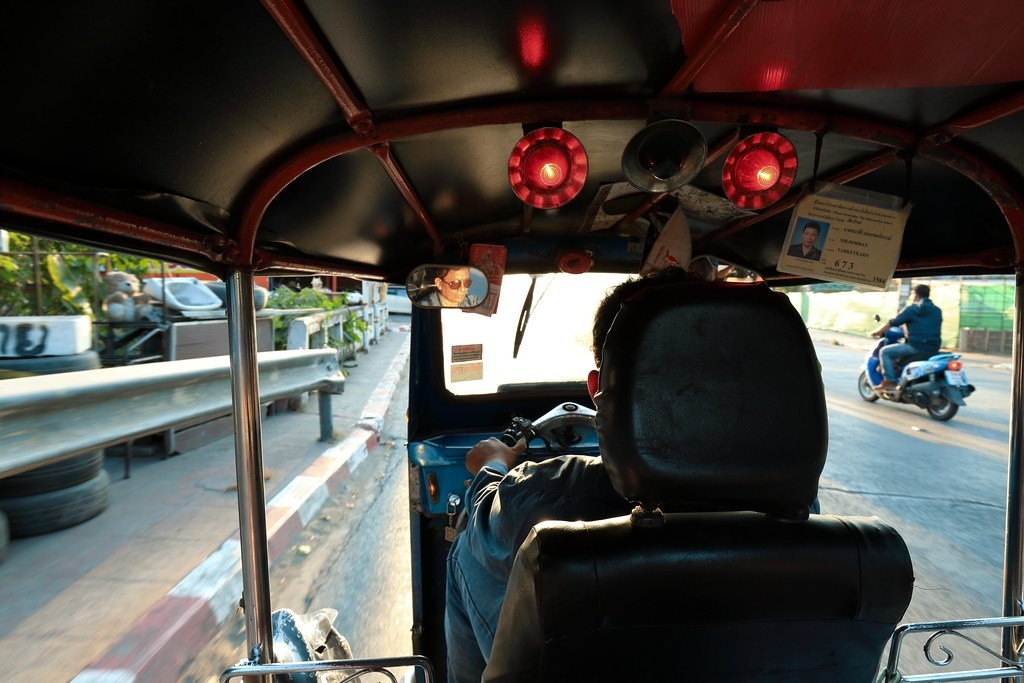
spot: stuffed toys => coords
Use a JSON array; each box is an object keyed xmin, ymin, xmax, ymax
[{"xmin": 103, "ymin": 271, "xmax": 153, "ymax": 321}]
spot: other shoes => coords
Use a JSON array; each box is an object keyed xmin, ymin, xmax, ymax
[{"xmin": 872, "ymin": 381, "xmax": 896, "ymax": 390}]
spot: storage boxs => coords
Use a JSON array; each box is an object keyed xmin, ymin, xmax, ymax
[{"xmin": 0, "ymin": 315, "xmax": 92, "ymax": 359}]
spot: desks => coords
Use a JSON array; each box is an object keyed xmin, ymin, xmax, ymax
[{"xmin": 93, "ymin": 319, "xmax": 171, "ymax": 478}]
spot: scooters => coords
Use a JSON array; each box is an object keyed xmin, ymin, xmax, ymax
[{"xmin": 857, "ymin": 314, "xmax": 976, "ymax": 421}]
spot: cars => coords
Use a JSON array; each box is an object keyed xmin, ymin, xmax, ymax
[{"xmin": 386, "ymin": 284, "xmax": 412, "ymax": 316}]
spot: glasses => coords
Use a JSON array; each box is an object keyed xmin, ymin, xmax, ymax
[{"xmin": 441, "ymin": 279, "xmax": 472, "ymax": 290}]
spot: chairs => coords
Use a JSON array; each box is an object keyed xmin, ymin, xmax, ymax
[{"xmin": 480, "ymin": 281, "xmax": 917, "ymax": 683}]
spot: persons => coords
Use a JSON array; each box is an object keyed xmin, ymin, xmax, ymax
[
  {"xmin": 443, "ymin": 268, "xmax": 821, "ymax": 683},
  {"xmin": 415, "ymin": 267, "xmax": 482, "ymax": 307},
  {"xmin": 871, "ymin": 284, "xmax": 943, "ymax": 390},
  {"xmin": 788, "ymin": 221, "xmax": 821, "ymax": 262}
]
